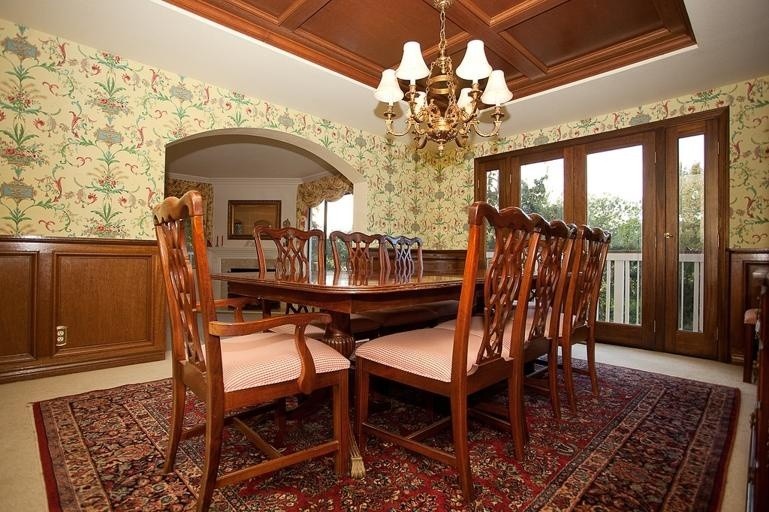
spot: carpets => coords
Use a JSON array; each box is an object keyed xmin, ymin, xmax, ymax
[{"xmin": 31, "ymin": 354, "xmax": 743, "ymax": 512}]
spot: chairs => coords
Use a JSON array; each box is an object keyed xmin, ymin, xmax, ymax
[
  {"xmin": 434, "ymin": 213, "xmax": 577, "ymax": 441},
  {"xmin": 353, "ymin": 201, "xmax": 547, "ymax": 503},
  {"xmin": 151, "ymin": 190, "xmax": 351, "ymax": 512},
  {"xmin": 497, "ymin": 224, "xmax": 611, "ymax": 418},
  {"xmin": 252, "ymin": 224, "xmax": 383, "ymax": 338},
  {"xmin": 329, "ymin": 230, "xmax": 435, "ymax": 332},
  {"xmin": 384, "ymin": 235, "xmax": 459, "ymax": 323}
]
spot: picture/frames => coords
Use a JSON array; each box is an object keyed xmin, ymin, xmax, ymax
[{"xmin": 227, "ymin": 199, "xmax": 282, "ymax": 240}]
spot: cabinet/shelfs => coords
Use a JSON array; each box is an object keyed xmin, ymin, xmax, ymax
[
  {"xmin": 718, "ymin": 248, "xmax": 769, "ymax": 365},
  {"xmin": 1, "ymin": 234, "xmax": 165, "ymax": 383}
]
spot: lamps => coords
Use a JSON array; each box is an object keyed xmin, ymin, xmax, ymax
[{"xmin": 373, "ymin": 0, "xmax": 513, "ymax": 157}]
